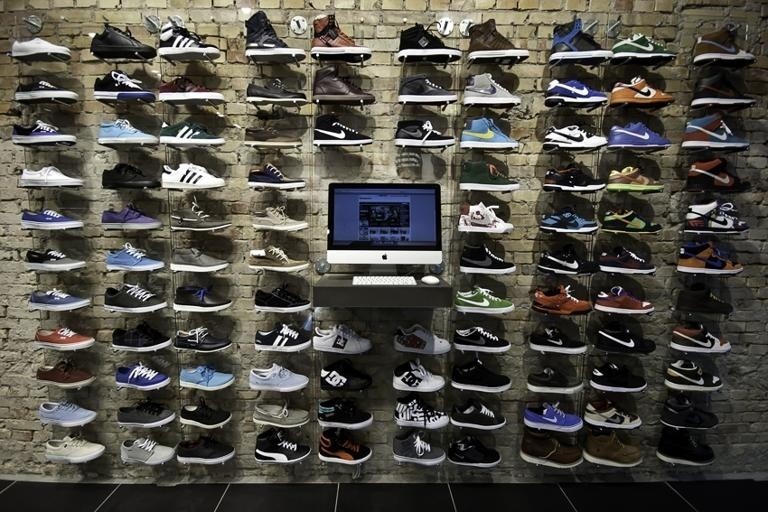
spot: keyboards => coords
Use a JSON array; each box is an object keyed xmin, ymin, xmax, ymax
[{"xmin": 352, "ymin": 272, "xmax": 418, "ymax": 287}]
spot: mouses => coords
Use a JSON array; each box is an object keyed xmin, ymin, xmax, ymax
[{"xmin": 421, "ymin": 273, "xmax": 442, "ymax": 287}]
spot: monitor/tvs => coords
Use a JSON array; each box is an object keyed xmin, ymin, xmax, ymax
[{"xmin": 327, "ymin": 182, "xmax": 443, "ymax": 264}]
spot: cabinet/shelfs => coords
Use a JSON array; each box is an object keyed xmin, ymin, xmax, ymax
[{"xmin": 12, "ymin": 10, "xmax": 757, "ymax": 468}]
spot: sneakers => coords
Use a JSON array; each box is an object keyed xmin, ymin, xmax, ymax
[
  {"xmin": 249, "ymin": 207, "xmax": 312, "ymax": 314},
  {"xmin": 656, "ymin": 433, "xmax": 715, "ymax": 467},
  {"xmin": 10, "ymin": 19, "xmax": 235, "ymax": 430},
  {"xmin": 247, "ymin": 163, "xmax": 305, "ymax": 189},
  {"xmin": 583, "ymin": 432, "xmax": 643, "ymax": 468},
  {"xmin": 456, "ymin": 202, "xmax": 516, "ymax": 314},
  {"xmin": 176, "ymin": 439, "xmax": 235, "ymax": 465},
  {"xmin": 521, "ymin": 15, "xmax": 755, "ymax": 429},
  {"xmin": 46, "ymin": 436, "xmax": 105, "ymax": 464},
  {"xmin": 458, "ymin": 158, "xmax": 520, "ymax": 191},
  {"xmin": 446, "ymin": 434, "xmax": 501, "ymax": 468},
  {"xmin": 254, "ymin": 433, "xmax": 312, "ymax": 464},
  {"xmin": 318, "ymin": 431, "xmax": 372, "ymax": 465},
  {"xmin": 249, "ymin": 326, "xmax": 514, "ymax": 431},
  {"xmin": 519, "ymin": 431, "xmax": 583, "ymax": 469},
  {"xmin": 244, "ymin": 13, "xmax": 524, "ymax": 150},
  {"xmin": 392, "ymin": 433, "xmax": 446, "ymax": 466},
  {"xmin": 120, "ymin": 438, "xmax": 174, "ymax": 466}
]
